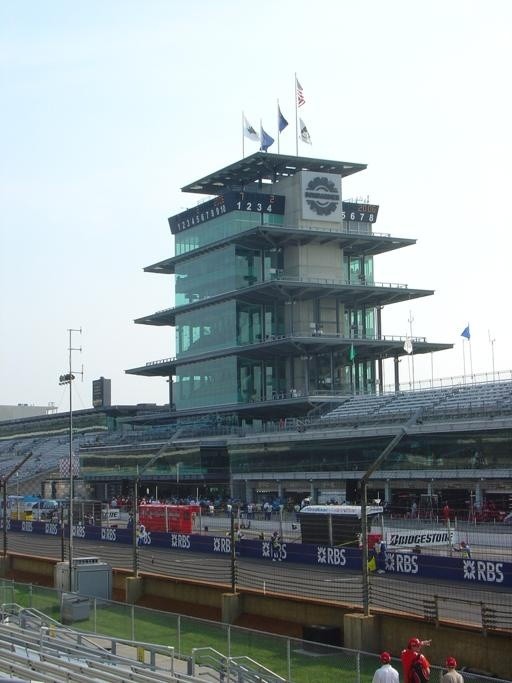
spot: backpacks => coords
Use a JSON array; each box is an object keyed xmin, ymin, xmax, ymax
[{"xmin": 408, "ymin": 654, "xmax": 431, "ymax": 683}]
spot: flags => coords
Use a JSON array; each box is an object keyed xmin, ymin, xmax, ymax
[
  {"xmin": 277, "ymin": 103, "xmax": 288, "ymax": 133},
  {"xmin": 295, "ymin": 76, "xmax": 307, "ymax": 108},
  {"xmin": 241, "ymin": 113, "xmax": 260, "ymax": 141},
  {"xmin": 460, "ymin": 326, "xmax": 470, "ymax": 340},
  {"xmin": 299, "ymin": 116, "xmax": 313, "ymax": 146},
  {"xmin": 258, "ymin": 127, "xmax": 274, "ymax": 150}
]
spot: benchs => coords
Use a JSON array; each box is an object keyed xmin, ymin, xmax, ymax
[
  {"xmin": 102, "ymin": 416, "xmax": 312, "ymax": 441},
  {"xmin": 319, "ymin": 379, "xmax": 512, "ymax": 421},
  {"xmin": 0, "ymin": 432, "xmax": 98, "ymax": 488},
  {"xmin": 0, "ymin": 621, "xmax": 205, "ymax": 682}
]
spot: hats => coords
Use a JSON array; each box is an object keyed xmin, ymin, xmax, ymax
[
  {"xmin": 379, "ymin": 652, "xmax": 392, "ymax": 664},
  {"xmin": 407, "ymin": 637, "xmax": 421, "ymax": 646},
  {"xmin": 445, "ymin": 657, "xmax": 457, "ymax": 668}
]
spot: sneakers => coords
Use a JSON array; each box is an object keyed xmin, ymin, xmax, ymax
[{"xmin": 271, "ymin": 557, "xmax": 281, "ymax": 561}]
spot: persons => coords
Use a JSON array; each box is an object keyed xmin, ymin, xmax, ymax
[
  {"xmin": 407, "ymin": 500, "xmax": 476, "ymax": 522},
  {"xmin": 80, "ymin": 494, "xmax": 217, "ymax": 523},
  {"xmin": 326, "ymin": 499, "xmax": 390, "ymax": 513},
  {"xmin": 400, "ymin": 637, "xmax": 432, "ymax": 683},
  {"xmin": 454, "ymin": 541, "xmax": 471, "ymax": 557},
  {"xmin": 440, "ymin": 656, "xmax": 463, "ymax": 683},
  {"xmin": 372, "ymin": 536, "xmax": 386, "ymax": 573},
  {"xmin": 224, "ymin": 496, "xmax": 314, "ymax": 522},
  {"xmin": 229, "ymin": 525, "xmax": 246, "ymax": 555},
  {"xmin": 372, "ymin": 651, "xmax": 399, "ymax": 682},
  {"xmin": 270, "ymin": 531, "xmax": 283, "ymax": 562}
]
